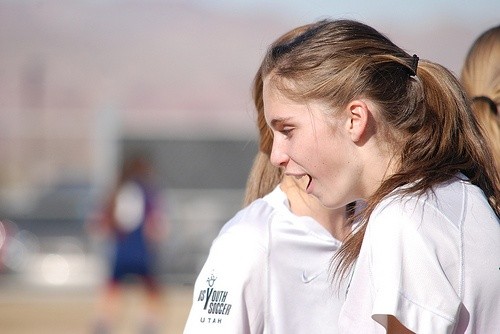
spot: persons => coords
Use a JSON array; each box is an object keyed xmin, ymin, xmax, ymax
[
  {"xmin": 459, "ymin": 26, "xmax": 500, "ymax": 194},
  {"xmin": 262, "ymin": 20, "xmax": 500, "ymax": 334},
  {"xmin": 182, "ymin": 65, "xmax": 370, "ymax": 334},
  {"xmin": 90, "ymin": 148, "xmax": 175, "ymax": 334}
]
